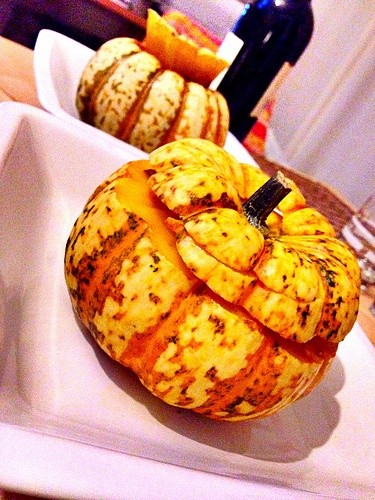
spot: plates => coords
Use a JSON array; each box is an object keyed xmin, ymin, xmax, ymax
[
  {"xmin": 32, "ymin": 27, "xmax": 260, "ymax": 172},
  {"xmin": 0, "ymin": 97, "xmax": 375, "ymax": 499}
]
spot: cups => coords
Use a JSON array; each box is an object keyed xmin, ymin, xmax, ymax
[{"xmin": 335, "ymin": 194, "xmax": 375, "ymax": 294}]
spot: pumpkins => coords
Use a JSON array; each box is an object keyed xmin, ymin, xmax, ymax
[
  {"xmin": 75, "ymin": 4, "xmax": 232, "ymax": 154},
  {"xmin": 62, "ymin": 136, "xmax": 363, "ymax": 423}
]
